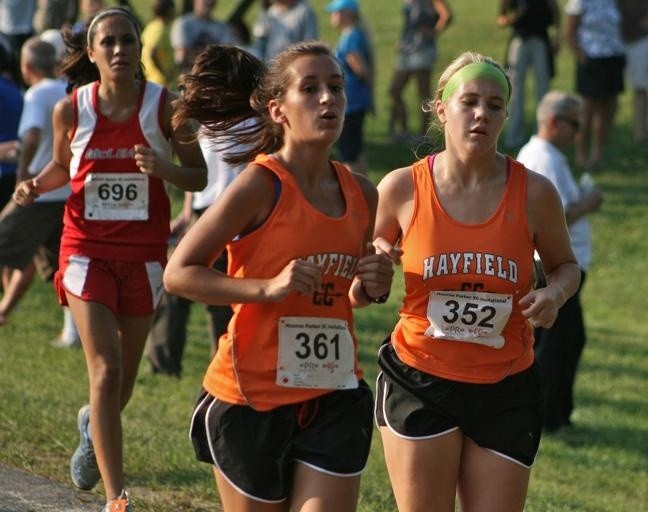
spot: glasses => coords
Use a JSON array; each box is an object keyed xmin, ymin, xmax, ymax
[{"xmin": 554, "ymin": 114, "xmax": 580, "ymax": 129}]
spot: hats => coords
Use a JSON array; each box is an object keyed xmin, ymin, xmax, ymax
[{"xmin": 324, "ymin": 0, "xmax": 359, "ymax": 13}]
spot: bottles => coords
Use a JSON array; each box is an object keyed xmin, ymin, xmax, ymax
[{"xmin": 579, "ymin": 171, "xmax": 594, "ymax": 195}]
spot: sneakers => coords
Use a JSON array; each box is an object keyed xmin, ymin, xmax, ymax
[{"xmin": 69, "ymin": 403, "xmax": 102, "ymax": 491}]
